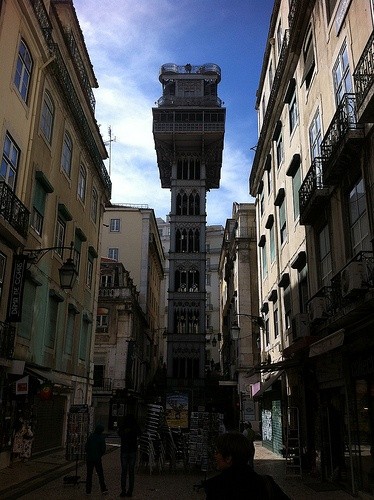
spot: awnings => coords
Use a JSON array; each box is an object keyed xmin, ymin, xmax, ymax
[
  {"xmin": 24, "ymin": 366, "xmax": 73, "ymax": 388},
  {"xmin": 253, "ymin": 370, "xmax": 286, "ymax": 399}
]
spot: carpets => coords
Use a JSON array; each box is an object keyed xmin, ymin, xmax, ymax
[{"xmin": 305, "ymin": 483, "xmax": 343, "ymax": 492}]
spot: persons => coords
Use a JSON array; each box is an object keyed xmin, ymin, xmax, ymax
[
  {"xmin": 84, "ymin": 425, "xmax": 110, "ymax": 495},
  {"xmin": 243, "ymin": 420, "xmax": 255, "ymax": 470},
  {"xmin": 201, "ymin": 432, "xmax": 289, "ymax": 500},
  {"xmin": 119, "ymin": 413, "xmax": 142, "ymax": 497}
]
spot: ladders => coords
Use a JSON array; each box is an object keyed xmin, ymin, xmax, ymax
[{"xmin": 284, "ymin": 405, "xmax": 303, "ymax": 483}]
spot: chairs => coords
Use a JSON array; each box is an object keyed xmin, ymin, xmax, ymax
[{"xmin": 138, "ymin": 404, "xmax": 178, "ymax": 477}]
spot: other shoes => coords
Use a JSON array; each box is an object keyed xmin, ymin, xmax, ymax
[
  {"xmin": 126, "ymin": 492, "xmax": 133, "ymax": 497},
  {"xmin": 120, "ymin": 491, "xmax": 126, "ymax": 496},
  {"xmin": 100, "ymin": 488, "xmax": 108, "ymax": 493},
  {"xmin": 86, "ymin": 491, "xmax": 91, "ymax": 494}
]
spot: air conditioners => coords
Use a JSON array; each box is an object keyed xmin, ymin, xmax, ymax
[
  {"xmin": 340, "ymin": 262, "xmax": 368, "ymax": 298},
  {"xmin": 307, "ymin": 297, "xmax": 327, "ymax": 325},
  {"xmin": 292, "ymin": 313, "xmax": 311, "ymax": 342}
]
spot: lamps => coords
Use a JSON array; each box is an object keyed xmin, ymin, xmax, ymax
[
  {"xmin": 230, "ymin": 311, "xmax": 265, "ymax": 341},
  {"xmin": 23, "ymin": 241, "xmax": 78, "ymax": 292},
  {"xmin": 212, "ymin": 334, "xmax": 224, "ymax": 347}
]
